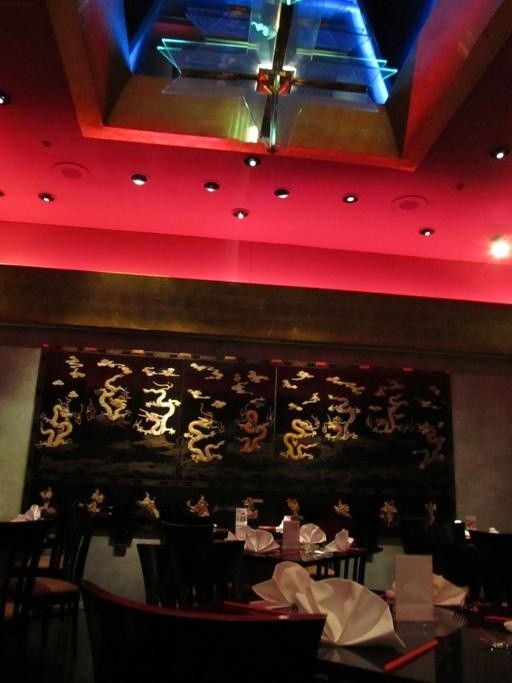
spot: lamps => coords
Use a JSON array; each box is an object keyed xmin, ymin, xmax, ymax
[
  {"xmin": 491, "ymin": 145, "xmax": 507, "ymax": 162},
  {"xmin": 1, "ymin": 94, "xmax": 11, "ymax": 104},
  {"xmin": 131, "ymin": 155, "xmax": 289, "ymax": 219}
]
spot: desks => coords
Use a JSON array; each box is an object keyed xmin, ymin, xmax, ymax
[{"xmin": 314, "ymin": 616, "xmax": 510, "ymax": 683}]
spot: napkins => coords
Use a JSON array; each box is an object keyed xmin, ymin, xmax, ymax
[
  {"xmin": 387, "ymin": 572, "xmax": 464, "ymax": 607},
  {"xmin": 256, "ymin": 561, "xmax": 403, "ymax": 652}
]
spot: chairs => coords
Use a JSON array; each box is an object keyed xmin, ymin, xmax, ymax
[
  {"xmin": 30, "ymin": 519, "xmax": 89, "ymax": 655},
  {"xmin": 1, "ymin": 509, "xmax": 510, "ymax": 593},
  {"xmin": 79, "ymin": 579, "xmax": 325, "ymax": 679},
  {"xmin": 2, "ymin": 519, "xmax": 46, "ymax": 658}
]
